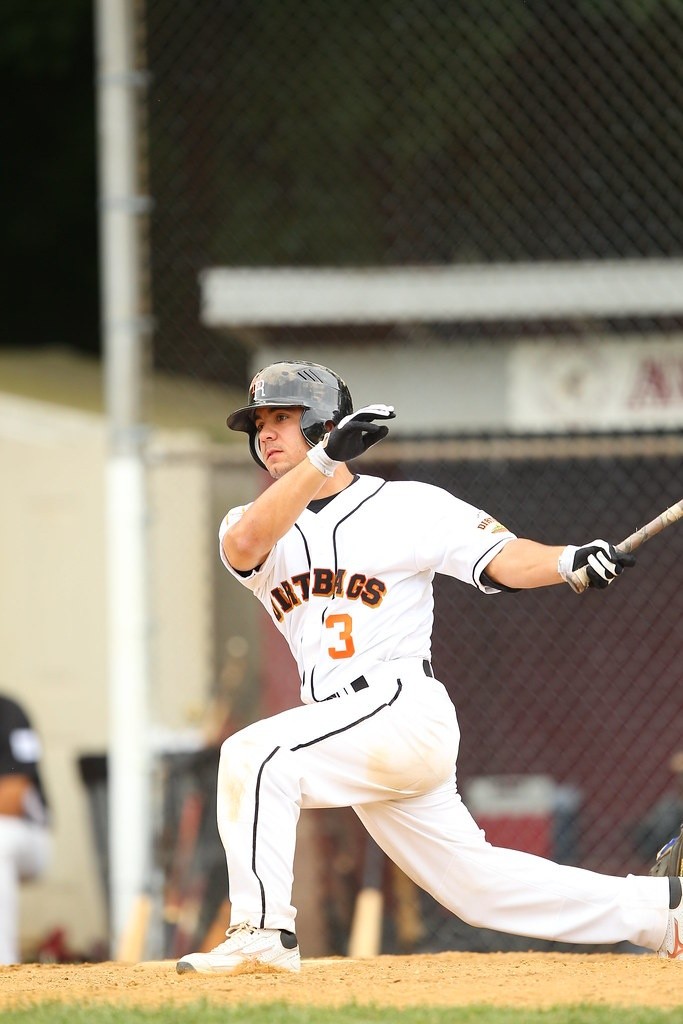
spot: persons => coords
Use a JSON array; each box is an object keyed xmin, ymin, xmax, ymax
[
  {"xmin": 0, "ymin": 697, "xmax": 246, "ymax": 969},
  {"xmin": 178, "ymin": 361, "xmax": 683, "ymax": 978}
]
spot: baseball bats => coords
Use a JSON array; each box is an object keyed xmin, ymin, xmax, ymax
[{"xmin": 563, "ymin": 499, "xmax": 683, "ymax": 596}]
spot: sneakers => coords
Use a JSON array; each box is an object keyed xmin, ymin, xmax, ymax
[
  {"xmin": 657, "ymin": 876, "xmax": 683, "ymax": 960},
  {"xmin": 176, "ymin": 923, "xmax": 300, "ymax": 976}
]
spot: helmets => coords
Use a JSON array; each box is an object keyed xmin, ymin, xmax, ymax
[{"xmin": 226, "ymin": 360, "xmax": 353, "ymax": 470}]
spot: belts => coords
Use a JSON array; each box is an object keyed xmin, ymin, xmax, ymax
[{"xmin": 322, "ymin": 660, "xmax": 432, "ymax": 701}]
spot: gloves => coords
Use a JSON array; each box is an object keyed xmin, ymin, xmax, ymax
[
  {"xmin": 558, "ymin": 539, "xmax": 635, "ymax": 592},
  {"xmin": 307, "ymin": 404, "xmax": 395, "ymax": 478}
]
